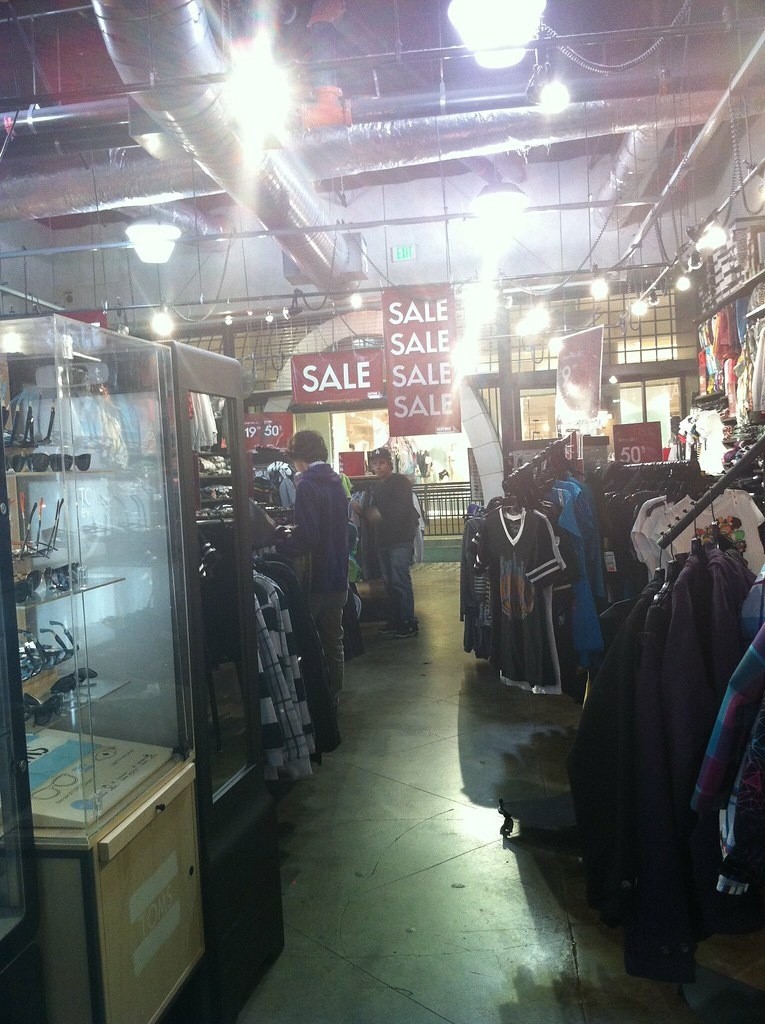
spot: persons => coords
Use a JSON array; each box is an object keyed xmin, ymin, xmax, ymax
[
  {"xmin": 275, "ymin": 430, "xmax": 352, "ymax": 712},
  {"xmin": 351, "ymin": 449, "xmax": 420, "ymax": 638}
]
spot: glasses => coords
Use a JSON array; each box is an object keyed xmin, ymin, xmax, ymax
[
  {"xmin": 11, "ymin": 453, "xmax": 50, "ymax": 472},
  {"xmin": 43, "ymin": 561, "xmax": 82, "ymax": 589},
  {"xmin": 49, "ymin": 667, "xmax": 98, "ymax": 694},
  {"xmin": 29, "ymin": 693, "xmax": 65, "ymax": 725},
  {"xmin": 51, "ymin": 453, "xmax": 91, "ymax": 472},
  {"xmin": 7, "ymin": 491, "xmax": 64, "ymax": 565},
  {"xmin": 16, "ymin": 620, "xmax": 80, "ymax": 682},
  {"xmin": 13, "ymin": 569, "xmax": 43, "ymax": 603},
  {"xmin": 22, "ymin": 692, "xmax": 40, "ymax": 721},
  {"xmin": 0, "ymin": 399, "xmax": 56, "ymax": 448}
]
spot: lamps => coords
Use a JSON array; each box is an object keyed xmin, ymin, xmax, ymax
[
  {"xmin": 283, "ymin": 306, "xmax": 303, "ymax": 320},
  {"xmin": 265, "ymin": 310, "xmax": 273, "ymax": 322},
  {"xmin": 525, "ymin": 82, "xmax": 569, "ymax": 113}
]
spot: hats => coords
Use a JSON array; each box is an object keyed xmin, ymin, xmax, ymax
[{"xmin": 370, "ymin": 448, "xmax": 391, "ymax": 460}]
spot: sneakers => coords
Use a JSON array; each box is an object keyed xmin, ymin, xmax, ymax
[
  {"xmin": 393, "ymin": 614, "xmax": 419, "ymax": 637},
  {"xmin": 376, "ymin": 618, "xmax": 398, "ymax": 633}
]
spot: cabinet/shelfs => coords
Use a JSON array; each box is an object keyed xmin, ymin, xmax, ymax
[
  {"xmin": 155, "ymin": 340, "xmax": 283, "ymax": 1024},
  {"xmin": 692, "ymin": 269, "xmax": 765, "ymax": 325},
  {"xmin": 0, "ymin": 314, "xmax": 200, "ymax": 1024},
  {"xmin": 0, "ymin": 420, "xmax": 50, "ymax": 1024}
]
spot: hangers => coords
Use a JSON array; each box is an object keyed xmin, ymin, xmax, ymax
[
  {"xmin": 599, "ymin": 460, "xmax": 753, "ymax": 607},
  {"xmin": 201, "ymin": 480, "xmax": 376, "ymax": 570},
  {"xmin": 502, "ymin": 439, "xmax": 588, "ymax": 515}
]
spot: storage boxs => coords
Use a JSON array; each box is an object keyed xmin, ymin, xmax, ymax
[{"xmin": 696, "ymin": 217, "xmax": 765, "ymax": 312}]
